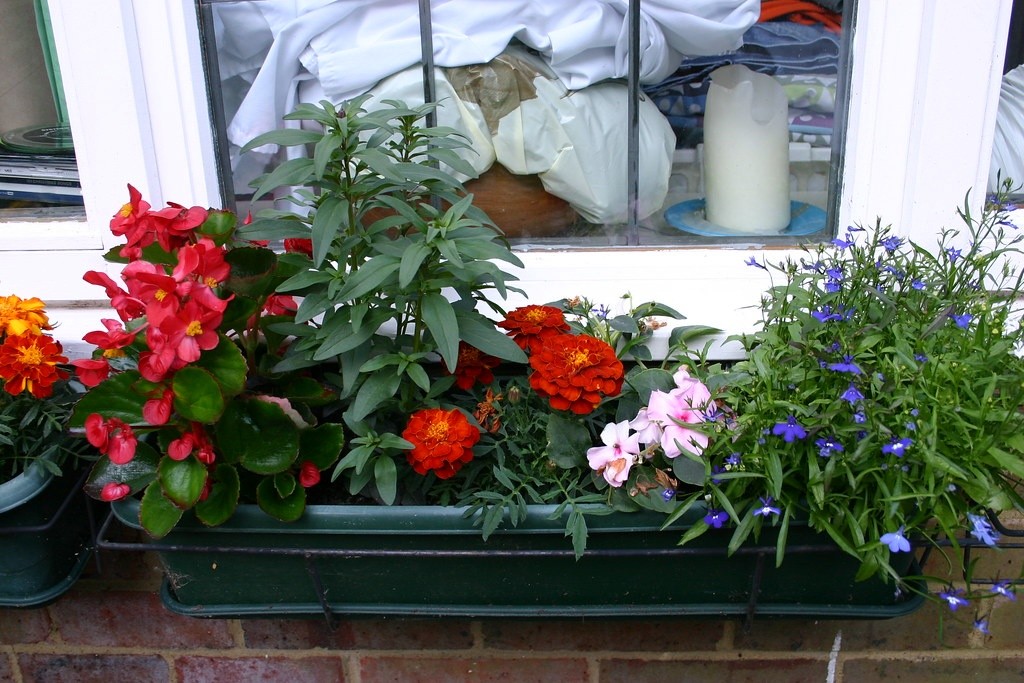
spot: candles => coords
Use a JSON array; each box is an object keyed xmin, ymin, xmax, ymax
[{"xmin": 702, "ymin": 65, "xmax": 792, "ymax": 233}]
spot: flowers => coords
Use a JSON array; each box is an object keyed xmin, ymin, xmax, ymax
[{"xmin": 0, "ymin": 95, "xmax": 1024, "ymax": 650}]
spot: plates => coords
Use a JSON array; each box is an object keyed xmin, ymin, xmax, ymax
[{"xmin": 664, "ymin": 199, "xmax": 827, "ymax": 236}]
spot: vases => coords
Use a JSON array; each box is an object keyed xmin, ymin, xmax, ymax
[
  {"xmin": 0, "ymin": 382, "xmax": 103, "ymax": 609},
  {"xmin": 109, "ymin": 432, "xmax": 926, "ymax": 620}
]
